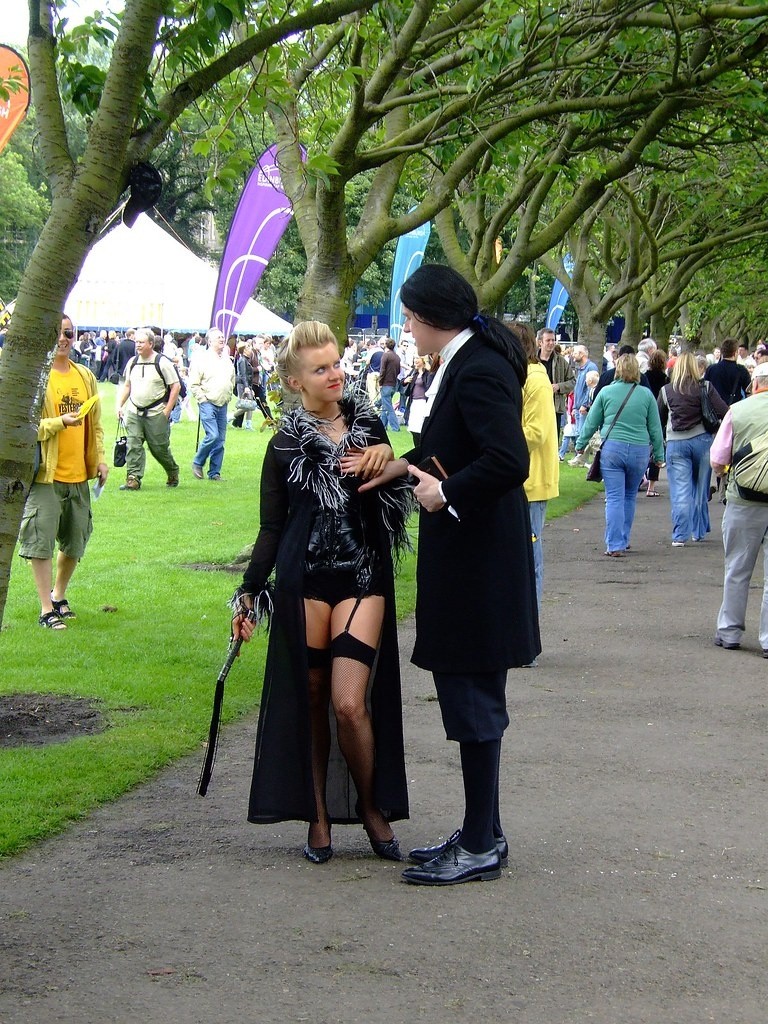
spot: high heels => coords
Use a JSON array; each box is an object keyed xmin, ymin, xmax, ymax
[
  {"xmin": 355, "ymin": 798, "xmax": 402, "ymax": 861},
  {"xmin": 303, "ymin": 814, "xmax": 333, "ymax": 864}
]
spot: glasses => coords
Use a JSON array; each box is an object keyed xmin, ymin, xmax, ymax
[{"xmin": 64, "ymin": 330, "xmax": 73, "ymax": 338}]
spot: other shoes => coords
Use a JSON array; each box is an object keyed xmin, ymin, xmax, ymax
[
  {"xmin": 713, "ymin": 632, "xmax": 739, "ymax": 649},
  {"xmin": 558, "ymin": 450, "xmax": 728, "ymax": 556},
  {"xmin": 763, "ymin": 649, "xmax": 768, "ymax": 658}
]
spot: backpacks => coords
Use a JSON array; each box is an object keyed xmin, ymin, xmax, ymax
[
  {"xmin": 733, "ymin": 430, "xmax": 768, "ymax": 503},
  {"xmin": 130, "ymin": 351, "xmax": 186, "ymax": 410}
]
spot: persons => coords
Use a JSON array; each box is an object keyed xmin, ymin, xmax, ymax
[
  {"xmin": 227, "ymin": 334, "xmax": 276, "ymax": 431},
  {"xmin": 116, "ymin": 328, "xmax": 182, "ymax": 490},
  {"xmin": 380, "ymin": 339, "xmax": 400, "ymax": 432},
  {"xmin": 655, "ymin": 352, "xmax": 728, "ymax": 548},
  {"xmin": 231, "ymin": 319, "xmax": 410, "ymax": 864},
  {"xmin": 71, "ymin": 328, "xmax": 136, "ymax": 383},
  {"xmin": 152, "ymin": 335, "xmax": 187, "ymax": 422},
  {"xmin": 188, "ymin": 327, "xmax": 235, "ymax": 481},
  {"xmin": 505, "ymin": 321, "xmax": 559, "ymax": 667},
  {"xmin": 341, "ymin": 337, "xmax": 434, "ymax": 415},
  {"xmin": 559, "ymin": 346, "xmax": 600, "ymax": 467},
  {"xmin": 164, "ymin": 330, "xmax": 207, "ymax": 359},
  {"xmin": 0, "ymin": 323, "xmax": 10, "ymax": 355},
  {"xmin": 342, "ymin": 264, "xmax": 541, "ymax": 886},
  {"xmin": 400, "ymin": 353, "xmax": 435, "ymax": 449},
  {"xmin": 710, "ymin": 362, "xmax": 768, "ymax": 658},
  {"xmin": 594, "ymin": 342, "xmax": 768, "ymax": 496},
  {"xmin": 574, "ymin": 355, "xmax": 664, "ymax": 557},
  {"xmin": 14, "ymin": 312, "xmax": 109, "ymax": 631},
  {"xmin": 536, "ymin": 327, "xmax": 577, "ymax": 438}
]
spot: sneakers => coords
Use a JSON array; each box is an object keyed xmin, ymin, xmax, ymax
[
  {"xmin": 193, "ymin": 465, "xmax": 204, "ymax": 479},
  {"xmin": 166, "ymin": 474, "xmax": 178, "ymax": 488},
  {"xmin": 120, "ymin": 479, "xmax": 140, "ymax": 490},
  {"xmin": 209, "ymin": 474, "xmax": 226, "ymax": 482}
]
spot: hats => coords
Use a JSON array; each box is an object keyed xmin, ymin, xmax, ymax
[{"xmin": 746, "ymin": 362, "xmax": 768, "ymax": 392}]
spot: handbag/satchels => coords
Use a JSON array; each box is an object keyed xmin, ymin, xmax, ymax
[
  {"xmin": 586, "ymin": 450, "xmax": 602, "ymax": 483},
  {"xmin": 404, "ymin": 370, "xmax": 418, "ymax": 396},
  {"xmin": 728, "ymin": 395, "xmax": 739, "ymax": 406},
  {"xmin": 236, "ymin": 391, "xmax": 257, "ymax": 411},
  {"xmin": 114, "ymin": 417, "xmax": 127, "ymax": 467},
  {"xmin": 110, "ymin": 372, "xmax": 119, "ymax": 385},
  {"xmin": 699, "ymin": 377, "xmax": 720, "ymax": 435}
]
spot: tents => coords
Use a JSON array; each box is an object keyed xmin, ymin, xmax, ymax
[{"xmin": 64, "ymin": 212, "xmax": 293, "ymax": 337}]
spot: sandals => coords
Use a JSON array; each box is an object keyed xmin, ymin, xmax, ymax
[
  {"xmin": 50, "ymin": 590, "xmax": 76, "ymax": 620},
  {"xmin": 38, "ymin": 609, "xmax": 67, "ymax": 630}
]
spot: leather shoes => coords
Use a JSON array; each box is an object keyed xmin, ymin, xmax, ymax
[
  {"xmin": 401, "ymin": 843, "xmax": 502, "ymax": 886},
  {"xmin": 408, "ymin": 828, "xmax": 508, "ymax": 867}
]
